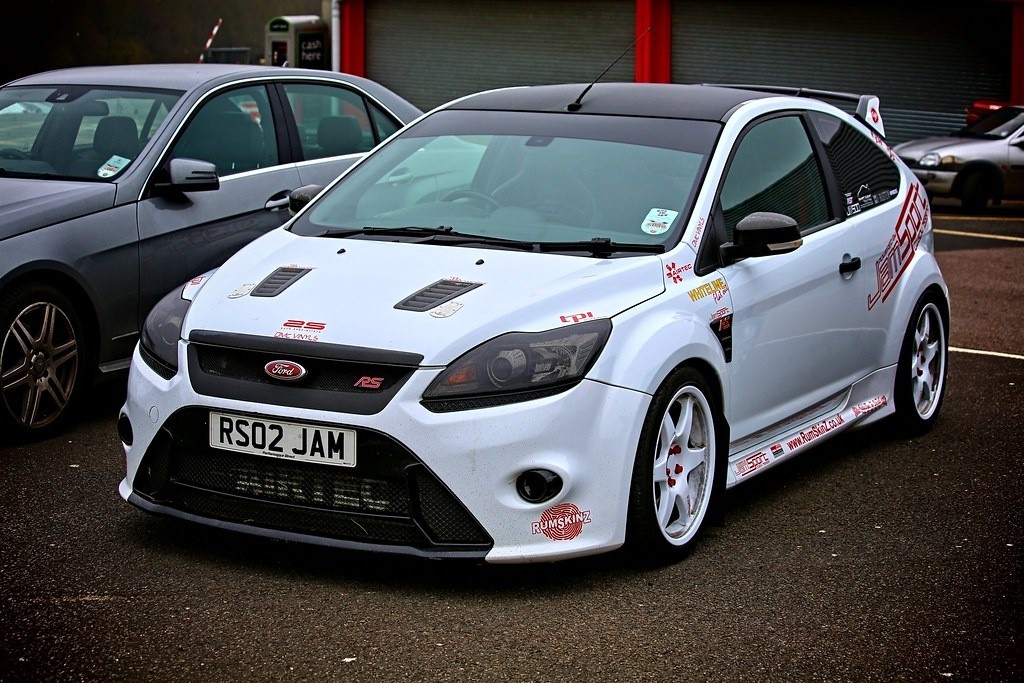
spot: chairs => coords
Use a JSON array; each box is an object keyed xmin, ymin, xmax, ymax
[
  {"xmin": 195, "ymin": 114, "xmax": 261, "ymax": 173},
  {"xmin": 94, "ymin": 117, "xmax": 137, "ymax": 170}
]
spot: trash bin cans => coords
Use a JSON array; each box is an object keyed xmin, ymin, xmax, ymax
[
  {"xmin": 264, "ymin": 15, "xmax": 330, "ymax": 70},
  {"xmin": 202, "ymin": 48, "xmax": 250, "ymax": 64}
]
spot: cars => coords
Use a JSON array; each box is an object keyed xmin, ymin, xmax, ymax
[
  {"xmin": 892, "ymin": 106, "xmax": 1024, "ymax": 208},
  {"xmin": 117, "ymin": 84, "xmax": 951, "ymax": 566},
  {"xmin": 0, "ymin": 64, "xmax": 425, "ymax": 442}
]
